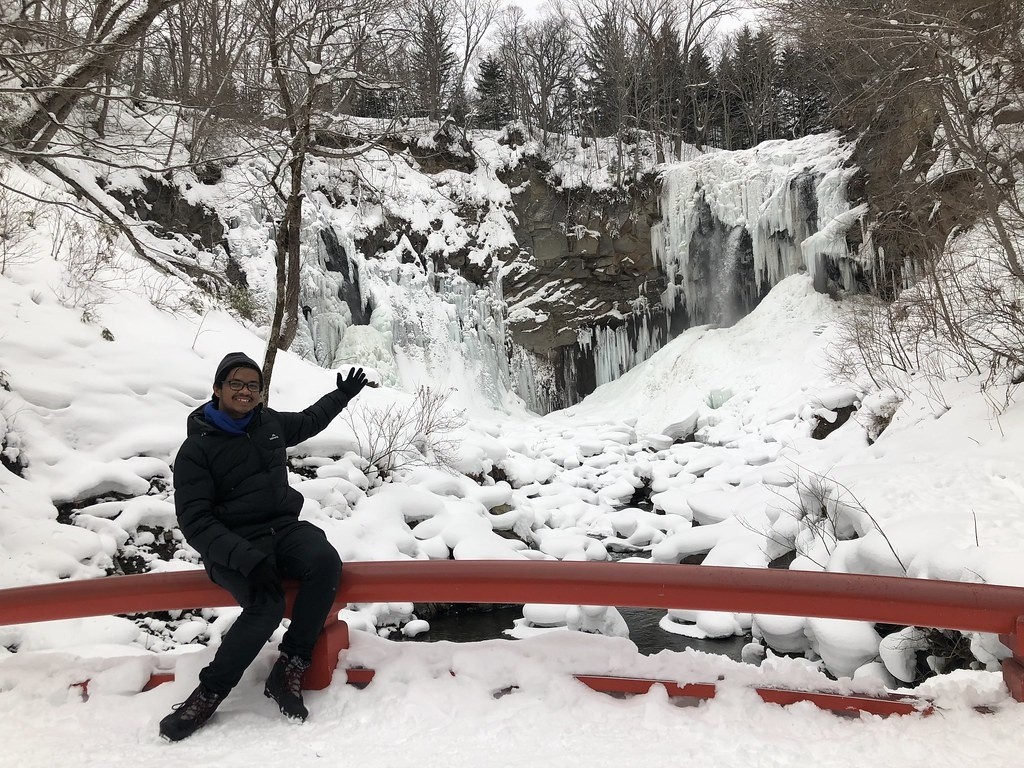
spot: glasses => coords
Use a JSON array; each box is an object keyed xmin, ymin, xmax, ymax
[{"xmin": 220, "ymin": 379, "xmax": 262, "ymax": 392}]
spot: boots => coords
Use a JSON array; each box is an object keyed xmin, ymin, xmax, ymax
[
  {"xmin": 264, "ymin": 653, "xmax": 308, "ymax": 721},
  {"xmin": 161, "ymin": 682, "xmax": 230, "ymax": 740}
]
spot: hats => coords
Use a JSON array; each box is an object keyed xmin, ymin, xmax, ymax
[{"xmin": 214, "ymin": 352, "xmax": 264, "ymax": 395}]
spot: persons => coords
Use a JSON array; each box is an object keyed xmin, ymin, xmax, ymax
[{"xmin": 158, "ymin": 352, "xmax": 368, "ymax": 742}]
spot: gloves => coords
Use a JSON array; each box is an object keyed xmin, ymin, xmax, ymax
[
  {"xmin": 337, "ymin": 368, "xmax": 368, "ymax": 403},
  {"xmin": 247, "ymin": 558, "xmax": 285, "ymax": 606}
]
